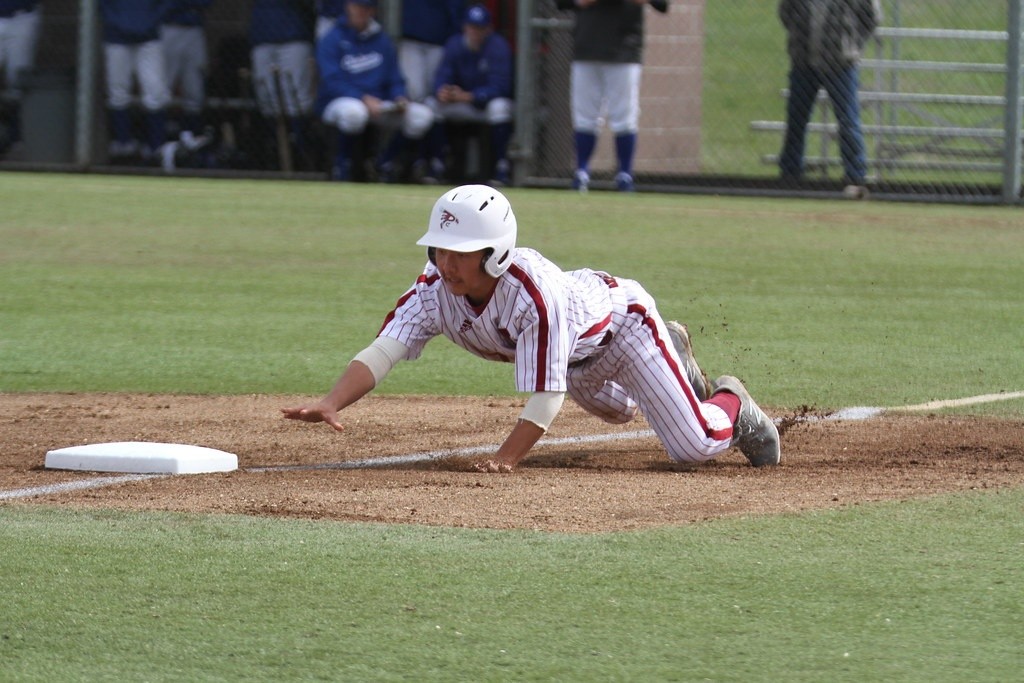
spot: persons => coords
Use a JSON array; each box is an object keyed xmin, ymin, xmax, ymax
[
  {"xmin": 0, "ymin": 1, "xmax": 47, "ymax": 94},
  {"xmin": 420, "ymin": 4, "xmax": 513, "ymax": 184},
  {"xmin": 774, "ymin": 0, "xmax": 879, "ymax": 198},
  {"xmin": 553, "ymin": 0, "xmax": 670, "ymax": 193},
  {"xmin": 97, "ymin": 0, "xmax": 210, "ymax": 171},
  {"xmin": 245, "ymin": 0, "xmax": 456, "ymax": 180},
  {"xmin": 276, "ymin": 184, "xmax": 779, "ymax": 476}
]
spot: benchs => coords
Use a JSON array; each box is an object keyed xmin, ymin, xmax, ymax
[{"xmin": 748, "ymin": 25, "xmax": 1023, "ymax": 187}]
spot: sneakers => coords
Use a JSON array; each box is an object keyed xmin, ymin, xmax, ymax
[
  {"xmin": 713, "ymin": 374, "xmax": 781, "ymax": 467},
  {"xmin": 664, "ymin": 320, "xmax": 710, "ymax": 403}
]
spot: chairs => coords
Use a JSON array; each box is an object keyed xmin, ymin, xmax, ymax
[{"xmin": 210, "ymin": 38, "xmax": 500, "ymax": 175}]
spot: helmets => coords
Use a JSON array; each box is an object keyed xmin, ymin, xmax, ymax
[{"xmin": 417, "ymin": 185, "xmax": 518, "ymax": 278}]
